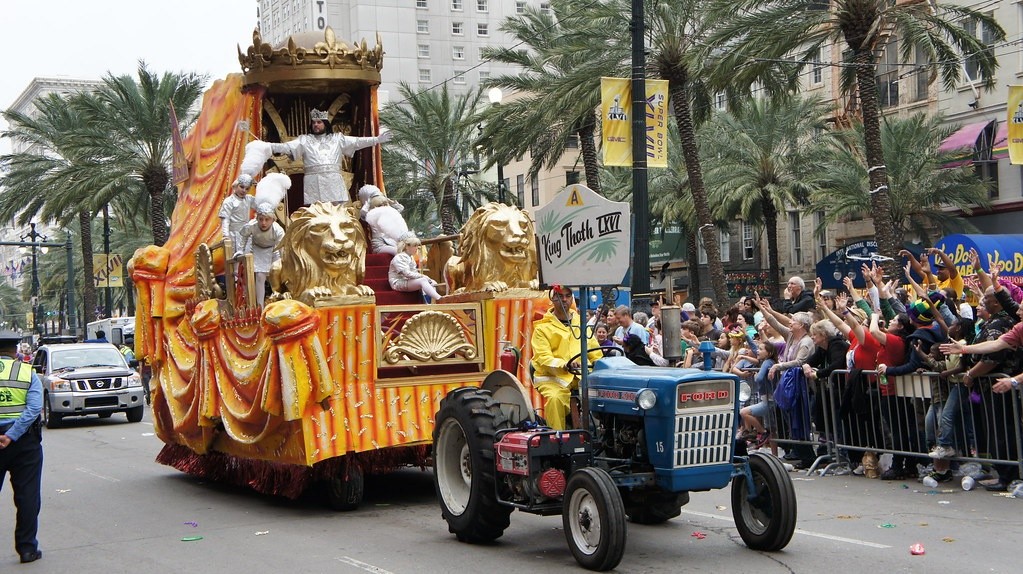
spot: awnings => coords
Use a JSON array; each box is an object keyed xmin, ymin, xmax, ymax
[
  {"xmin": 993, "ymin": 124, "xmax": 1010, "ymax": 159},
  {"xmin": 936, "ymin": 118, "xmax": 996, "ymax": 168}
]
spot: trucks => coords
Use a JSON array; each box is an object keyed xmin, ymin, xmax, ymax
[{"xmin": 78, "ymin": 317, "xmax": 136, "ymax": 348}]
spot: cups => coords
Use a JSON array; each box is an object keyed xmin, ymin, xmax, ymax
[
  {"xmin": 961, "ymin": 476, "xmax": 975, "ymax": 491},
  {"xmin": 923, "ymin": 476, "xmax": 938, "ymax": 488}
]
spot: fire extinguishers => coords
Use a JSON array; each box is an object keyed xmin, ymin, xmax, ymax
[{"xmin": 500, "ymin": 345, "xmax": 519, "ymax": 378}]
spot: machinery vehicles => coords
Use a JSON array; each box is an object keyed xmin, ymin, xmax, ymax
[{"xmin": 128, "ymin": 22, "xmax": 798, "ymax": 572}]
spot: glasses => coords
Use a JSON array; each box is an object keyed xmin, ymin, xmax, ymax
[{"xmin": 823, "ymin": 298, "xmax": 832, "ymax": 301}]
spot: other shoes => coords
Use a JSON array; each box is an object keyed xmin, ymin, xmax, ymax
[
  {"xmin": 19, "ymin": 549, "xmax": 41, "ymax": 563},
  {"xmin": 423, "ymin": 295, "xmax": 432, "ymax": 305},
  {"xmin": 734, "ymin": 423, "xmax": 1023, "ymax": 493}
]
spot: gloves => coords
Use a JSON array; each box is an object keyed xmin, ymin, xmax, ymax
[
  {"xmin": 374, "ymin": 129, "xmax": 395, "ymax": 144},
  {"xmin": 232, "ymin": 249, "xmax": 243, "ymax": 259}
]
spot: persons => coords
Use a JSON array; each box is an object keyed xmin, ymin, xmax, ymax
[
  {"xmin": 218, "ymin": 141, "xmax": 272, "ymax": 287},
  {"xmin": 531, "ymin": 286, "xmax": 603, "ymax": 433},
  {"xmin": 0, "ymin": 331, "xmax": 44, "ymax": 563},
  {"xmin": 120, "ymin": 339, "xmax": 152, "ymax": 405},
  {"xmin": 271, "ymin": 110, "xmax": 394, "ymax": 206},
  {"xmin": 676, "ymin": 247, "xmax": 1023, "ymax": 496},
  {"xmin": 84, "ymin": 331, "xmax": 109, "ymax": 344},
  {"xmin": 388, "ymin": 238, "xmax": 442, "ymax": 300},
  {"xmin": 586, "ymin": 294, "xmax": 694, "ymax": 367},
  {"xmin": 17, "ymin": 343, "xmax": 37, "ymax": 365},
  {"xmin": 234, "ymin": 173, "xmax": 291, "ymax": 311},
  {"xmin": 359, "ymin": 185, "xmax": 408, "ymax": 254}
]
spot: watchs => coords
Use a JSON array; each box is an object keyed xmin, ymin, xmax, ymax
[{"xmin": 1010, "ymin": 377, "xmax": 1018, "ymax": 387}]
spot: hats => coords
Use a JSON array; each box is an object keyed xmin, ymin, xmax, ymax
[
  {"xmin": 399, "ymin": 230, "xmax": 417, "ymax": 242},
  {"xmin": 964, "ymin": 285, "xmax": 972, "ymax": 289},
  {"xmin": 846, "ymin": 307, "xmax": 866, "ymax": 320},
  {"xmin": 681, "ymin": 303, "xmax": 696, "ymax": 312},
  {"xmin": 238, "ymin": 140, "xmax": 272, "ymax": 186},
  {"xmin": 358, "ymin": 185, "xmax": 382, "ymax": 204},
  {"xmin": 680, "ymin": 311, "xmax": 689, "ymax": 322},
  {"xmin": 905, "ymin": 292, "xmax": 945, "ymax": 325},
  {"xmin": 623, "ymin": 332, "xmax": 646, "ymax": 346},
  {"xmin": 933, "ymin": 260, "xmax": 946, "ymax": 268},
  {"xmin": 255, "ymin": 172, "xmax": 291, "ymax": 214},
  {"xmin": 819, "ymin": 290, "xmax": 835, "ymax": 299},
  {"xmin": 961, "ymin": 292, "xmax": 966, "ymax": 301},
  {"xmin": 125, "ymin": 338, "xmax": 134, "ymax": 344},
  {"xmin": 728, "ymin": 326, "xmax": 746, "ymax": 342},
  {"xmin": 0, "ymin": 331, "xmax": 22, "ymax": 348},
  {"xmin": 602, "ymin": 310, "xmax": 608, "ymax": 316},
  {"xmin": 649, "ymin": 295, "xmax": 666, "ymax": 306}
]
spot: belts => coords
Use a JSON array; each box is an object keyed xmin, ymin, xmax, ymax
[{"xmin": 0, "ymin": 421, "xmax": 42, "ymax": 432}]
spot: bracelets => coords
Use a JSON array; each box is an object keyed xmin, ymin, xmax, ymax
[
  {"xmin": 865, "ymin": 278, "xmax": 871, "ymax": 282},
  {"xmin": 994, "ymin": 284, "xmax": 1001, "ymax": 289},
  {"xmin": 962, "ymin": 345, "xmax": 966, "ymax": 354},
  {"xmin": 966, "ymin": 371, "xmax": 974, "ymax": 380},
  {"xmin": 976, "ymin": 267, "xmax": 983, "ymax": 274},
  {"xmin": 842, "ymin": 310, "xmax": 849, "ymax": 315},
  {"xmin": 937, "ymin": 249, "xmax": 943, "ymax": 255}
]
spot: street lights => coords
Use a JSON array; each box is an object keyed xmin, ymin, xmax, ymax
[{"xmin": 18, "ymin": 214, "xmax": 49, "ymax": 331}]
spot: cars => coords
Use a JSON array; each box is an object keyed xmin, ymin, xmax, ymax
[{"xmin": 16, "ymin": 343, "xmax": 145, "ymax": 429}]
